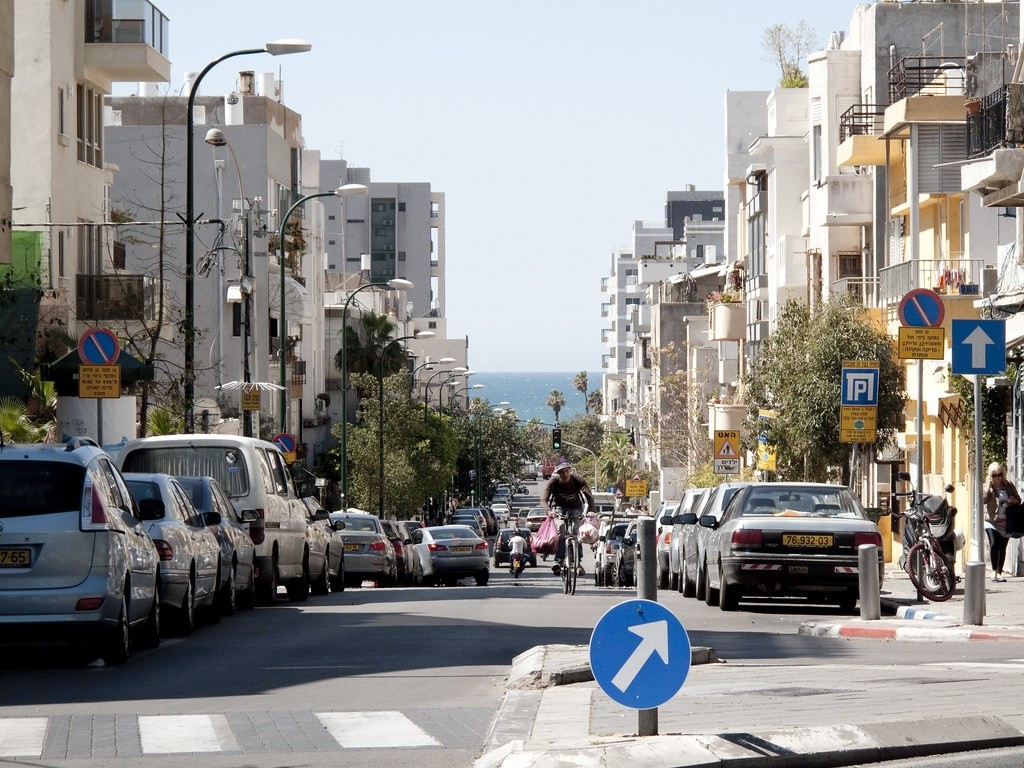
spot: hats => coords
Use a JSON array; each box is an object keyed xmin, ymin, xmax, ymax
[{"xmin": 554, "ymin": 462, "xmax": 571, "ymax": 472}]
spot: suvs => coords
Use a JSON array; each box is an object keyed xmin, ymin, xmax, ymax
[
  {"xmin": 491, "ymin": 529, "xmax": 537, "ymax": 567},
  {"xmin": 0, "ymin": 435, "xmax": 164, "ymax": 670}
]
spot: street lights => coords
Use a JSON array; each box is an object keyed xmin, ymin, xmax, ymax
[
  {"xmin": 380, "ymin": 330, "xmax": 437, "ymax": 521},
  {"xmin": 338, "ymin": 280, "xmax": 413, "ymax": 510},
  {"xmin": 409, "ymin": 358, "xmax": 511, "ymax": 520},
  {"xmin": 279, "ymin": 184, "xmax": 369, "ymax": 430},
  {"xmin": 185, "ymin": 41, "xmax": 316, "ymax": 430},
  {"xmin": 201, "ymin": 129, "xmax": 253, "ymax": 435}
]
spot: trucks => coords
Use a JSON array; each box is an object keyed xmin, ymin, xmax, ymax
[{"xmin": 521, "ymin": 459, "xmax": 558, "ymax": 481}]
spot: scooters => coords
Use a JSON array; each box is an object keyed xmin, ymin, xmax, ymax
[{"xmin": 513, "ymin": 483, "xmax": 529, "ymax": 495}]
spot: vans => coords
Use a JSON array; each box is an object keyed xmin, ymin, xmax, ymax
[
  {"xmin": 114, "ymin": 434, "xmax": 310, "ymax": 606},
  {"xmin": 582, "ymin": 492, "xmax": 616, "ymax": 518}
]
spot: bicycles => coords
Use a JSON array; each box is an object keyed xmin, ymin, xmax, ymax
[
  {"xmin": 553, "ymin": 513, "xmax": 587, "ymax": 595},
  {"xmin": 894, "ymin": 495, "xmax": 956, "ymax": 602}
]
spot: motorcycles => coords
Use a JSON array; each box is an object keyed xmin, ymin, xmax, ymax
[
  {"xmin": 892, "ymin": 472, "xmax": 958, "ymax": 594},
  {"xmin": 510, "ymin": 553, "xmax": 523, "ymax": 578}
]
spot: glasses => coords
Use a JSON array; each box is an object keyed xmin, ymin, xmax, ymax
[{"xmin": 992, "ymin": 474, "xmax": 1004, "ymax": 478}]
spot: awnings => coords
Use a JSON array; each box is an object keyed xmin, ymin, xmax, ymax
[
  {"xmin": 268, "ymin": 272, "xmax": 315, "ymax": 325},
  {"xmin": 227, "ymin": 284, "xmax": 241, "ymax": 303}
]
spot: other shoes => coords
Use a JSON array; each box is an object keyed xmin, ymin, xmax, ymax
[
  {"xmin": 578, "ymin": 563, "xmax": 585, "ymax": 575},
  {"xmin": 510, "ymin": 569, "xmax": 513, "ymax": 574},
  {"xmin": 991, "ymin": 572, "xmax": 1008, "ymax": 582},
  {"xmin": 552, "ymin": 562, "xmax": 561, "ymax": 576}
]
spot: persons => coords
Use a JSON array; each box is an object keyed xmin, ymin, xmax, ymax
[
  {"xmin": 541, "ymin": 459, "xmax": 596, "ymax": 576},
  {"xmin": 983, "ymin": 462, "xmax": 1021, "ymax": 582},
  {"xmin": 508, "ymin": 530, "xmax": 530, "ymax": 573}
]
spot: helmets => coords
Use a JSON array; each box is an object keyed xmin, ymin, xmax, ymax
[
  {"xmin": 954, "ymin": 529, "xmax": 966, "ymax": 551},
  {"xmin": 515, "ymin": 529, "xmax": 522, "ymax": 536}
]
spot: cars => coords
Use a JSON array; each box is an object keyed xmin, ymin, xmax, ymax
[
  {"xmin": 122, "ymin": 473, "xmax": 222, "ymax": 634},
  {"xmin": 515, "ymin": 507, "xmax": 548, "ymax": 531},
  {"xmin": 595, "ymin": 482, "xmax": 885, "ymax": 612},
  {"xmin": 305, "ymin": 484, "xmax": 510, "ymax": 588},
  {"xmin": 174, "ymin": 476, "xmax": 262, "ymax": 618}
]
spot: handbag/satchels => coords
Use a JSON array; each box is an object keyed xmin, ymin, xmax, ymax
[
  {"xmin": 994, "ymin": 500, "xmax": 1024, "ymax": 538},
  {"xmin": 577, "ymin": 521, "xmax": 599, "ymax": 543},
  {"xmin": 530, "ymin": 516, "xmax": 559, "ymax": 554}
]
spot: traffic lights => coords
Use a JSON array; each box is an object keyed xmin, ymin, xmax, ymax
[{"xmin": 552, "ymin": 429, "xmax": 562, "ymax": 449}]
